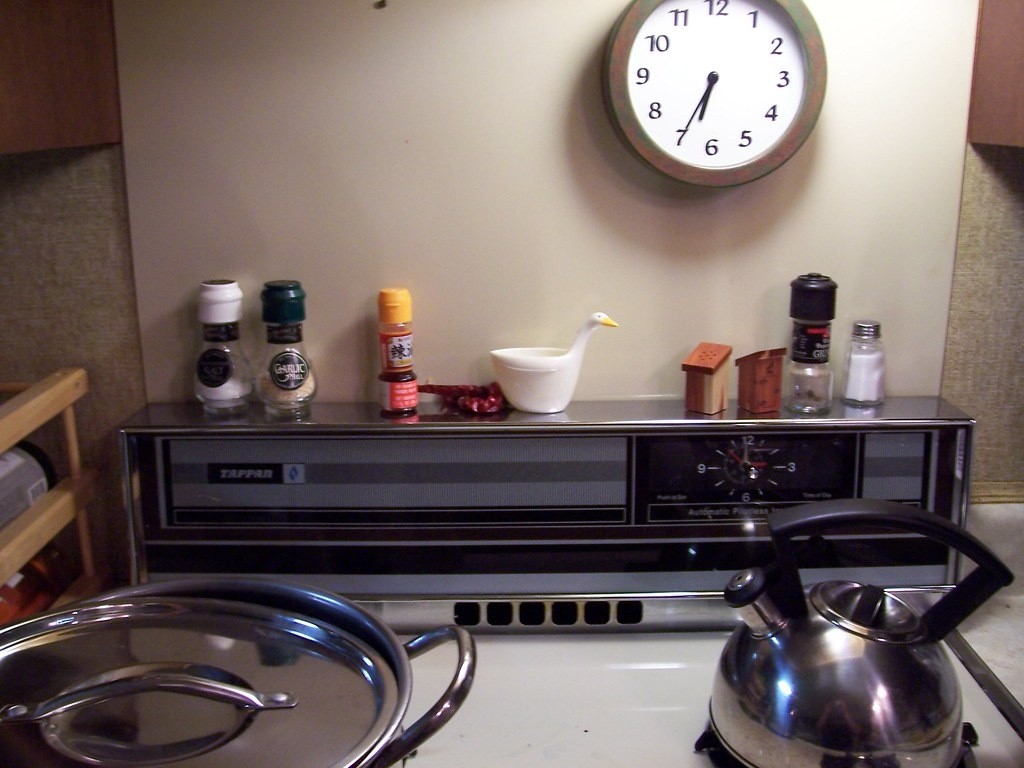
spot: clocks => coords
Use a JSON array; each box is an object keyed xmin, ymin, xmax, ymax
[{"xmin": 600, "ymin": 0, "xmax": 826, "ymax": 188}]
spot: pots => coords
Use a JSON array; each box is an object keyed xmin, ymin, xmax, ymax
[{"xmin": 0, "ymin": 576, "xmax": 477, "ymax": 768}]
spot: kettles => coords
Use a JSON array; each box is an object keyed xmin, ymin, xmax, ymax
[{"xmin": 706, "ymin": 498, "xmax": 1015, "ymax": 768}]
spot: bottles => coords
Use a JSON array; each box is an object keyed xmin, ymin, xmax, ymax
[
  {"xmin": 192, "ymin": 280, "xmax": 254, "ymax": 415},
  {"xmin": 786, "ymin": 273, "xmax": 837, "ymax": 414},
  {"xmin": 840, "ymin": 320, "xmax": 887, "ymax": 406},
  {"xmin": 252, "ymin": 280, "xmax": 317, "ymax": 416},
  {"xmin": 377, "ymin": 287, "xmax": 419, "ymax": 413}
]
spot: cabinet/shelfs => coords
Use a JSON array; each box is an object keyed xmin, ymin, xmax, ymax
[{"xmin": 0, "ymin": 366, "xmax": 102, "ymax": 636}]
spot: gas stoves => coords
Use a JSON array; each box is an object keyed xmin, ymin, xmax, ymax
[{"xmin": 117, "ymin": 392, "xmax": 1024, "ymax": 768}]
